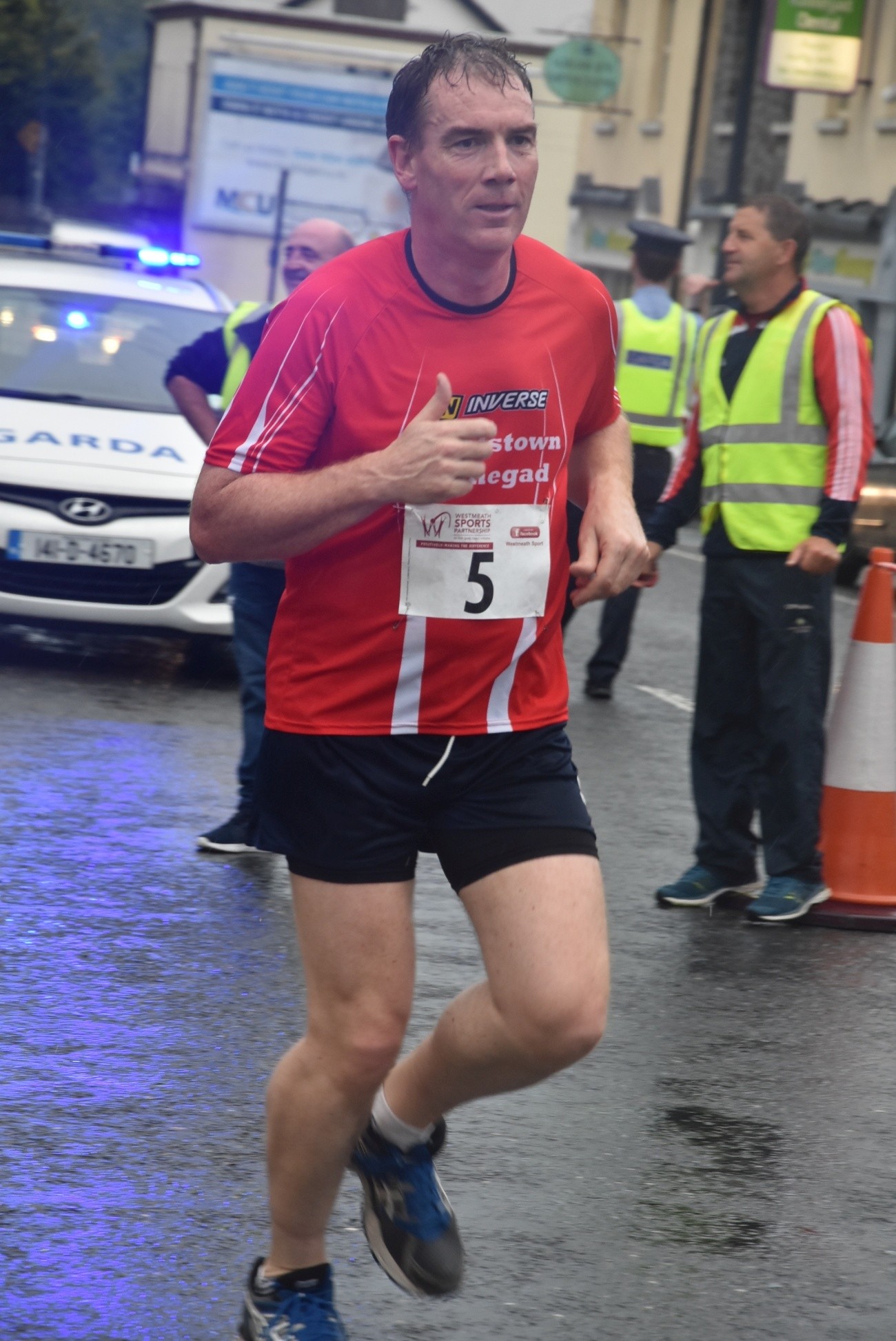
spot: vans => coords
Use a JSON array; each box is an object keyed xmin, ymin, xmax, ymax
[{"xmin": 0, "ymin": 230, "xmax": 244, "ymax": 678}]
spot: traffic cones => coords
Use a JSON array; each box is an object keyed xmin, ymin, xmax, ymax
[{"xmin": 798, "ymin": 542, "xmax": 896, "ymax": 931}]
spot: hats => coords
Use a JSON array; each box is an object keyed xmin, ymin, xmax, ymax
[{"xmin": 625, "ymin": 220, "xmax": 694, "ymax": 244}]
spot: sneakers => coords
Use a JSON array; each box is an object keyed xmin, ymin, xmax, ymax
[
  {"xmin": 585, "ymin": 678, "xmax": 613, "ymax": 699},
  {"xmin": 237, "ymin": 1257, "xmax": 347, "ymax": 1341},
  {"xmin": 198, "ymin": 807, "xmax": 271, "ymax": 853},
  {"xmin": 347, "ymin": 1112, "xmax": 464, "ymax": 1298},
  {"xmin": 654, "ymin": 862, "xmax": 763, "ymax": 905},
  {"xmin": 745, "ymin": 876, "xmax": 833, "ymax": 921}
]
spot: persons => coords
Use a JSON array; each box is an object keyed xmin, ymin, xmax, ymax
[
  {"xmin": 188, "ymin": 36, "xmax": 650, "ymax": 1340},
  {"xmin": 629, "ymin": 188, "xmax": 873, "ymax": 925},
  {"xmin": 160, "ymin": 218, "xmax": 357, "ymax": 853},
  {"xmin": 563, "ymin": 216, "xmax": 704, "ymax": 697}
]
